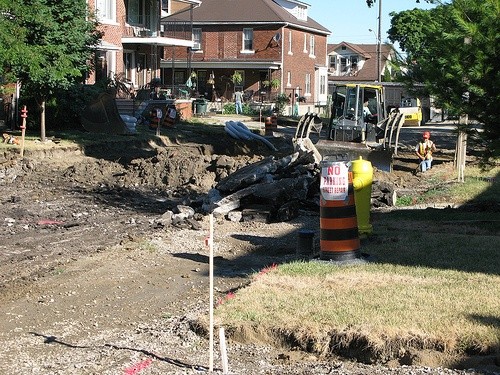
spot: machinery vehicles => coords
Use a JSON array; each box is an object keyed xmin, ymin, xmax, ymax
[
  {"xmin": 389, "ymin": 96, "xmax": 426, "ymax": 127},
  {"xmin": 314, "ymin": 83, "xmax": 394, "ymax": 177}
]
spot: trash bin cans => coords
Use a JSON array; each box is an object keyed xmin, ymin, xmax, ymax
[{"xmin": 194, "ymin": 98, "xmax": 208, "ymax": 115}]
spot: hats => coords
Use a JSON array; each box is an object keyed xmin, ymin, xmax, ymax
[{"xmin": 423, "ymin": 131, "xmax": 430, "ymax": 137}]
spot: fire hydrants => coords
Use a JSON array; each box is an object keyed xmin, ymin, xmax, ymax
[{"xmin": 350, "ymin": 155, "xmax": 374, "ymax": 237}]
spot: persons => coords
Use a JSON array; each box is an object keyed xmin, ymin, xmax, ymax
[
  {"xmin": 415, "ymin": 132, "xmax": 436, "ymax": 172},
  {"xmin": 234, "ymin": 88, "xmax": 243, "ymax": 114}
]
